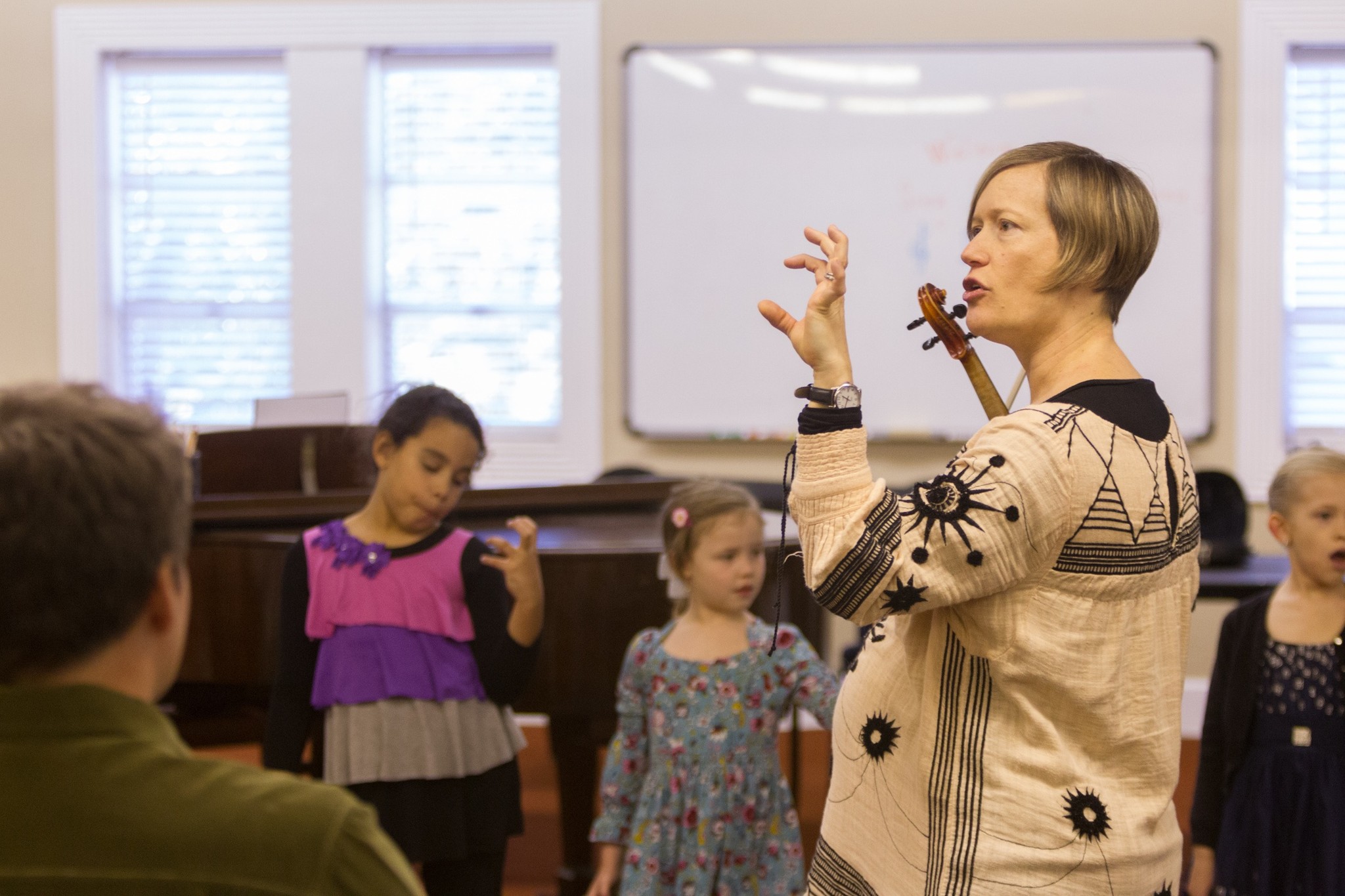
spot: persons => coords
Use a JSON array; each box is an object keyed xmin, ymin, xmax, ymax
[
  {"xmin": 757, "ymin": 141, "xmax": 1202, "ymax": 896},
  {"xmin": 1185, "ymin": 449, "xmax": 1345, "ymax": 896},
  {"xmin": 583, "ymin": 482, "xmax": 845, "ymax": 896},
  {"xmin": 0, "ymin": 383, "xmax": 424, "ymax": 896},
  {"xmin": 260, "ymin": 383, "xmax": 545, "ymax": 896}
]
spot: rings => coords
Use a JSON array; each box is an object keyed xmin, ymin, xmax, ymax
[{"xmin": 823, "ymin": 271, "xmax": 837, "ymax": 280}]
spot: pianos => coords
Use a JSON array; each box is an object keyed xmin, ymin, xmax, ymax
[{"xmin": 158, "ymin": 477, "xmax": 833, "ymax": 896}]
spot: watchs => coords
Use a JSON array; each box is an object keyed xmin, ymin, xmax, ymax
[{"xmin": 794, "ymin": 381, "xmax": 862, "ymax": 408}]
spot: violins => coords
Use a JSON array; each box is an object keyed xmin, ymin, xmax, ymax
[{"xmin": 906, "ymin": 279, "xmax": 1008, "ymax": 424}]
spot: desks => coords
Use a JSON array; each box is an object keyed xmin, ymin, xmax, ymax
[{"xmin": 1196, "ymin": 554, "xmax": 1292, "ymax": 605}]
